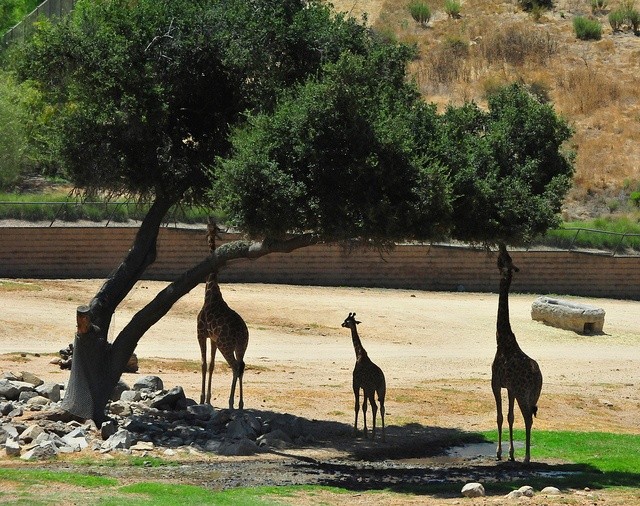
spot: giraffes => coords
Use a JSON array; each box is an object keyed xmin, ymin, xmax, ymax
[
  {"xmin": 341, "ymin": 312, "xmax": 386, "ymax": 443},
  {"xmin": 197, "ymin": 216, "xmax": 249, "ymax": 410},
  {"xmin": 491, "ymin": 242, "xmax": 542, "ymax": 466}
]
